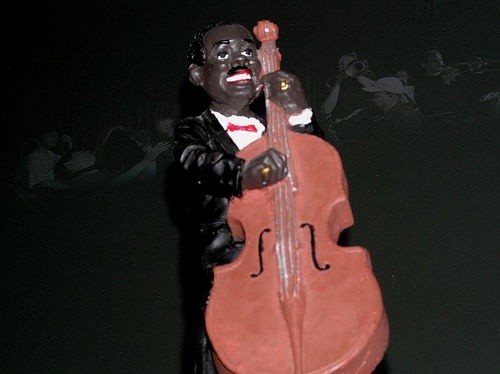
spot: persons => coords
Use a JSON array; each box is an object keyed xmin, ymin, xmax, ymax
[
  {"xmin": 8, "ymin": 115, "xmax": 176, "ymax": 208},
  {"xmin": 163, "ymin": 14, "xmax": 327, "ymax": 374},
  {"xmin": 307, "ymin": 45, "xmax": 500, "ymax": 170}
]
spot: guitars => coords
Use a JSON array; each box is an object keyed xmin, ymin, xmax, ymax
[{"xmin": 204, "ymin": 20, "xmax": 389, "ymax": 374}]
